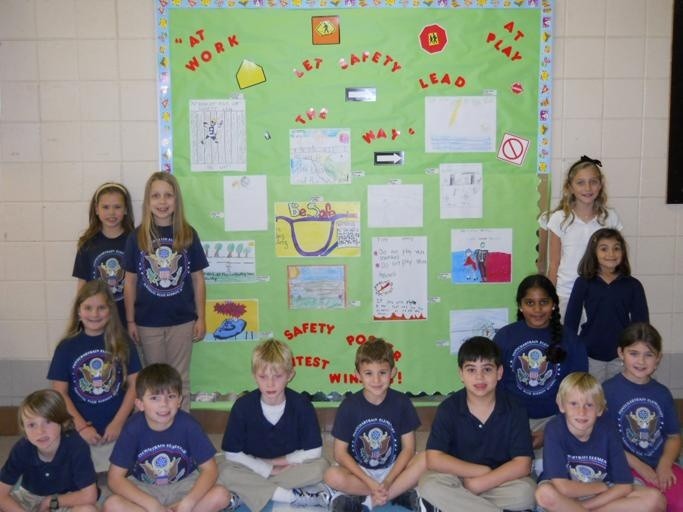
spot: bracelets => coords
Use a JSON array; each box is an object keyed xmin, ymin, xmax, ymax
[
  {"xmin": 76, "ymin": 422, "xmax": 94, "ymax": 432},
  {"xmin": 50, "ymin": 494, "xmax": 58, "ymax": 509},
  {"xmin": 127, "ymin": 321, "xmax": 135, "ymax": 324}
]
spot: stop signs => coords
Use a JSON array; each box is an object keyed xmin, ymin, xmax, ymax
[{"xmin": 495, "ymin": 130, "xmax": 531, "ymax": 170}]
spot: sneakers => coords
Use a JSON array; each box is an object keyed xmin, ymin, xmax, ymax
[
  {"xmin": 418, "ymin": 497, "xmax": 439, "ymax": 512},
  {"xmin": 223, "ymin": 490, "xmax": 240, "ymax": 511},
  {"xmin": 329, "ymin": 493, "xmax": 371, "ymax": 512},
  {"xmin": 290, "ymin": 483, "xmax": 331, "ymax": 508},
  {"xmin": 389, "ymin": 486, "xmax": 421, "ymax": 512}
]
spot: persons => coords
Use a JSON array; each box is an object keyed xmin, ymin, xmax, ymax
[
  {"xmin": 493, "ymin": 275, "xmax": 589, "ymax": 475},
  {"xmin": 71, "ymin": 183, "xmax": 136, "ymax": 333},
  {"xmin": 214, "ymin": 339, "xmax": 337, "ymax": 512},
  {"xmin": 602, "ymin": 322, "xmax": 683, "ymax": 512},
  {"xmin": 564, "ymin": 228, "xmax": 649, "ymax": 383},
  {"xmin": 103, "ymin": 362, "xmax": 231, "ymax": 512},
  {"xmin": 323, "ymin": 338, "xmax": 427, "ymax": 512},
  {"xmin": 538, "ymin": 210, "xmax": 548, "ymax": 277},
  {"xmin": 46, "ymin": 281, "xmax": 144, "ymax": 474},
  {"xmin": 0, "ymin": 388, "xmax": 102, "ymax": 512},
  {"xmin": 124, "ymin": 172, "xmax": 209, "ymax": 414},
  {"xmin": 534, "ymin": 372, "xmax": 667, "ymax": 512},
  {"xmin": 418, "ymin": 336, "xmax": 538, "ymax": 512},
  {"xmin": 546, "ymin": 156, "xmax": 623, "ymax": 336}
]
